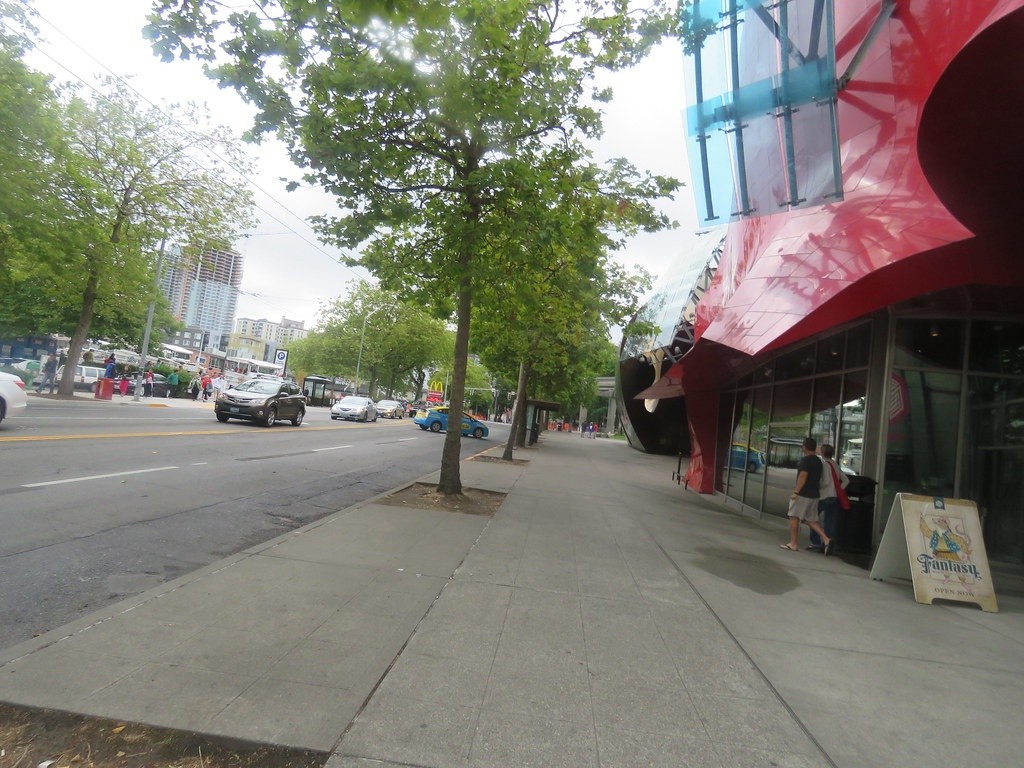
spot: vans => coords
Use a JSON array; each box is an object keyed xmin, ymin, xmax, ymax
[{"xmin": 54, "ymin": 364, "xmax": 107, "ymax": 394}]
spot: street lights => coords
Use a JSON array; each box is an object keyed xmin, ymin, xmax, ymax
[{"xmin": 353, "ymin": 303, "xmax": 396, "ymax": 398}]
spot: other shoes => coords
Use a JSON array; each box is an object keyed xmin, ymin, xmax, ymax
[
  {"xmin": 203, "ymin": 399, "xmax": 207, "ymax": 402},
  {"xmin": 805, "ymin": 545, "xmax": 822, "ymax": 553},
  {"xmin": 46, "ymin": 392, "xmax": 53, "ymax": 395},
  {"xmin": 35, "ymin": 388, "xmax": 42, "ymax": 394}
]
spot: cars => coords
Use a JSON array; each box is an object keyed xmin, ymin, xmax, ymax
[
  {"xmin": 413, "ymin": 405, "xmax": 489, "ymax": 439},
  {"xmin": 113, "ymin": 371, "xmax": 173, "ymax": 398},
  {"xmin": 0, "ymin": 371, "xmax": 28, "ymax": 423},
  {"xmin": 408, "ymin": 400, "xmax": 433, "ymax": 417},
  {"xmin": 330, "ymin": 396, "xmax": 378, "ymax": 422},
  {"xmin": 842, "ymin": 437, "xmax": 863, "ymax": 475},
  {"xmin": 726, "ymin": 443, "xmax": 766, "ymax": 473},
  {"xmin": 375, "ymin": 399, "xmax": 405, "ymax": 419},
  {"xmin": 0, "ymin": 357, "xmax": 53, "ymax": 387}
]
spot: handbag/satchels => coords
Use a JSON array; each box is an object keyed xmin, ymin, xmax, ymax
[
  {"xmin": 826, "ymin": 461, "xmax": 850, "ymax": 510},
  {"xmin": 126, "ymin": 371, "xmax": 135, "ymax": 380}
]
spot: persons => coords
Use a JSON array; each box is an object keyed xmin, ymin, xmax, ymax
[
  {"xmin": 85, "ymin": 349, "xmax": 94, "ymax": 362},
  {"xmin": 105, "ymin": 360, "xmax": 116, "ymax": 379},
  {"xmin": 166, "ymin": 370, "xmax": 179, "ymax": 397},
  {"xmin": 144, "ymin": 369, "xmax": 154, "ymax": 396},
  {"xmin": 304, "ymin": 387, "xmax": 309, "ymax": 396},
  {"xmin": 58, "ymin": 350, "xmax": 65, "ymax": 369},
  {"xmin": 188, "ymin": 371, "xmax": 226, "ymax": 402},
  {"xmin": 805, "ymin": 445, "xmax": 849, "ymax": 552},
  {"xmin": 106, "ymin": 353, "xmax": 115, "ymax": 363},
  {"xmin": 580, "ymin": 421, "xmax": 598, "ymax": 439},
  {"xmin": 119, "ymin": 377, "xmax": 128, "ymax": 396},
  {"xmin": 402, "ymin": 401, "xmax": 407, "ymax": 411},
  {"xmin": 35, "ymin": 352, "xmax": 57, "ymax": 394},
  {"xmin": 779, "ymin": 438, "xmax": 833, "ymax": 556},
  {"xmin": 234, "ymin": 367, "xmax": 247, "ymax": 374}
]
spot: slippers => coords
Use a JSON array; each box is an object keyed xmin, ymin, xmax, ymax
[
  {"xmin": 780, "ymin": 543, "xmax": 799, "ymax": 552},
  {"xmin": 824, "ymin": 539, "xmax": 833, "ymax": 556}
]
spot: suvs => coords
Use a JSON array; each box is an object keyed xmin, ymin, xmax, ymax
[{"xmin": 213, "ymin": 373, "xmax": 307, "ymax": 428}]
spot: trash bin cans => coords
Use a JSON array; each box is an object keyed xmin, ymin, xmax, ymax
[
  {"xmin": 534, "ymin": 423, "xmax": 539, "ymax": 444},
  {"xmin": 824, "ymin": 475, "xmax": 876, "ymax": 558},
  {"xmin": 95, "ymin": 376, "xmax": 114, "ymax": 401}
]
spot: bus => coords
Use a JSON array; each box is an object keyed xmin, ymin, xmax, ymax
[
  {"xmin": 221, "ymin": 356, "xmax": 283, "ymax": 390},
  {"xmin": 159, "ymin": 342, "xmax": 193, "ymax": 364}
]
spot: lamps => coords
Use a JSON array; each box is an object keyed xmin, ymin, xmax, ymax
[
  {"xmin": 831, "ymin": 346, "xmax": 841, "ymax": 356},
  {"xmin": 765, "ymin": 366, "xmax": 773, "ymax": 376},
  {"xmin": 930, "ymin": 322, "xmax": 939, "ymax": 337}
]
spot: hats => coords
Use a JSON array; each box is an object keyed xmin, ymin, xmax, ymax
[{"xmin": 204, "ymin": 375, "xmax": 211, "ymax": 378}]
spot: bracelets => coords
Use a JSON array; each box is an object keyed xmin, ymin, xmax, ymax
[{"xmin": 793, "ymin": 492, "xmax": 799, "ymax": 495}]
